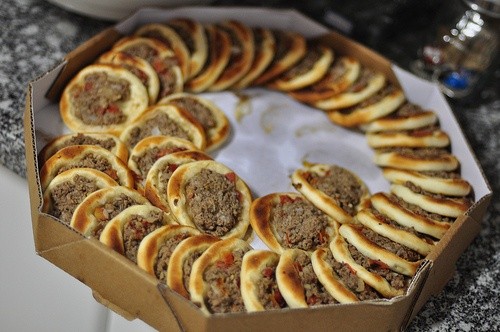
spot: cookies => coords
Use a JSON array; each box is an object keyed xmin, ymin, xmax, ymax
[{"xmin": 37, "ymin": 14, "xmax": 473, "ymax": 314}]
